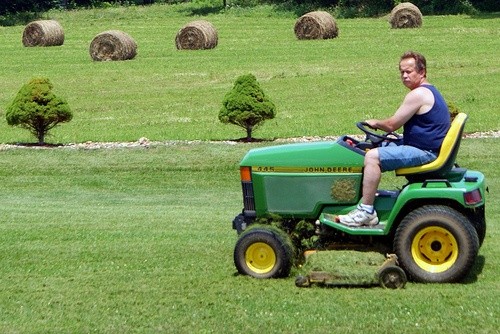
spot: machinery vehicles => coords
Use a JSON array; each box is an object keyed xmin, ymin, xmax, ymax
[{"xmin": 232, "ymin": 113, "xmax": 489, "ymax": 290}]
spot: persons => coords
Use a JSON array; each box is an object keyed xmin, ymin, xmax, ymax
[{"xmin": 338, "ymin": 51, "xmax": 451, "ymax": 228}]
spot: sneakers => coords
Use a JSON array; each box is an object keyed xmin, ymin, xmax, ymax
[{"xmin": 334, "ymin": 204, "xmax": 379, "ymax": 227}]
